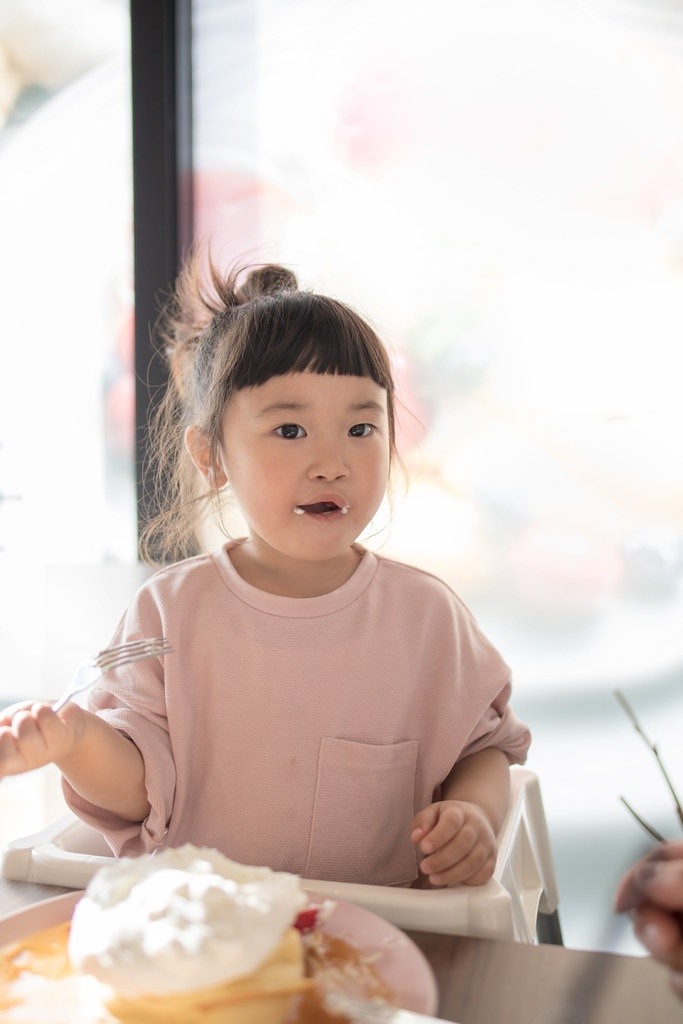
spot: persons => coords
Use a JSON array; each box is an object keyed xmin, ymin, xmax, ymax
[
  {"xmin": 0, "ymin": 254, "xmax": 530, "ymax": 893},
  {"xmin": 612, "ymin": 843, "xmax": 683, "ymax": 1005}
]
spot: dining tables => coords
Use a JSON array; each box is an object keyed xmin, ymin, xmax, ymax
[{"xmin": 0, "ymin": 877, "xmax": 683, "ymax": 1024}]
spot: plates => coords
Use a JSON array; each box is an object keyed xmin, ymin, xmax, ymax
[{"xmin": 1, "ymin": 889, "xmax": 439, "ymax": 1024}]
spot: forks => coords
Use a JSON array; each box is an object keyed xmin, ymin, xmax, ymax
[{"xmin": 49, "ymin": 640, "xmax": 177, "ymax": 711}]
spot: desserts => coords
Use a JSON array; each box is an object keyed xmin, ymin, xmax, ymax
[{"xmin": 66, "ymin": 842, "xmax": 322, "ymax": 1024}]
